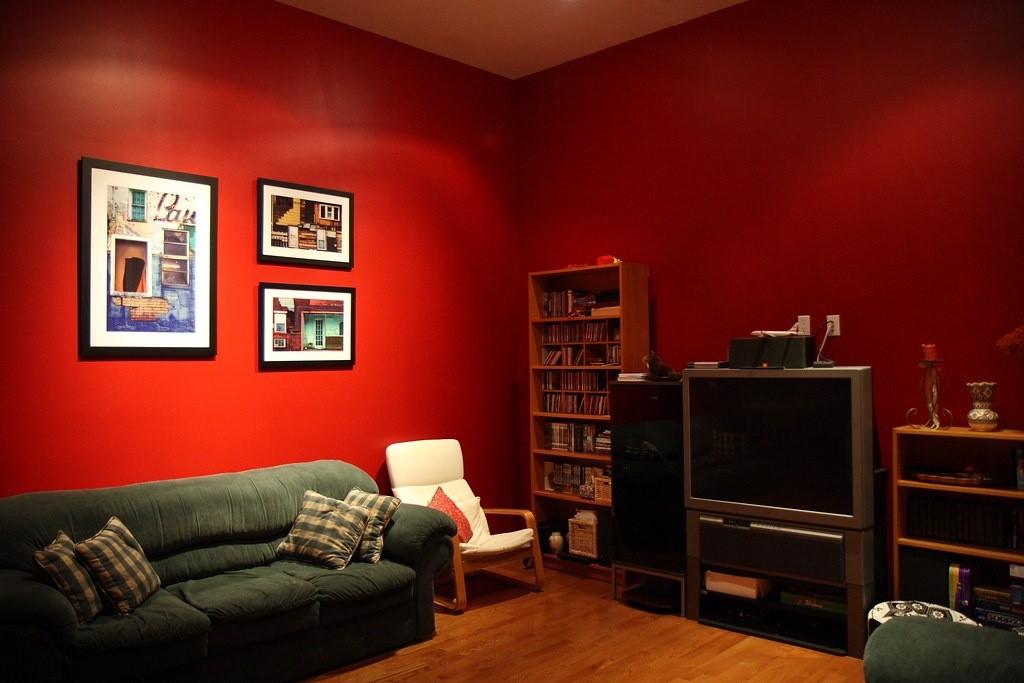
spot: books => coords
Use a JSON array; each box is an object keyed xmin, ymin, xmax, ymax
[
  {"xmin": 542, "ymin": 320, "xmax": 621, "ymax": 367},
  {"xmin": 544, "ymin": 461, "xmax": 604, "ymax": 499},
  {"xmin": 539, "ymin": 370, "xmax": 607, "ymax": 415},
  {"xmin": 544, "ymin": 422, "xmax": 611, "ymax": 455},
  {"xmin": 540, "ymin": 287, "xmax": 621, "ymax": 318},
  {"xmin": 974, "ymin": 583, "xmax": 1024, "ymax": 628}
]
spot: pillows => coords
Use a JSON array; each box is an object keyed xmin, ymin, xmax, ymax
[
  {"xmin": 72, "ymin": 515, "xmax": 161, "ymax": 616},
  {"xmin": 342, "ymin": 486, "xmax": 402, "ymax": 565},
  {"xmin": 275, "ymin": 490, "xmax": 371, "ymax": 570},
  {"xmin": 427, "ymin": 487, "xmax": 473, "ymax": 544},
  {"xmin": 33, "ymin": 529, "xmax": 107, "ymax": 626}
]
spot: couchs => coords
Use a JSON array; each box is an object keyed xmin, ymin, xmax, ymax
[{"xmin": 0, "ymin": 459, "xmax": 459, "ymax": 683}]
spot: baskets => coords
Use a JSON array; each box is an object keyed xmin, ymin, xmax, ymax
[
  {"xmin": 595, "ymin": 476, "xmax": 612, "ymax": 502},
  {"xmin": 568, "ymin": 518, "xmax": 606, "ymax": 560}
]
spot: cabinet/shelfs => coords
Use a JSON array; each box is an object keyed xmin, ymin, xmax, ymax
[
  {"xmin": 685, "ymin": 510, "xmax": 885, "ymax": 658},
  {"xmin": 527, "ymin": 263, "xmax": 650, "ymax": 580},
  {"xmin": 892, "ymin": 424, "xmax": 1024, "ymax": 634}
]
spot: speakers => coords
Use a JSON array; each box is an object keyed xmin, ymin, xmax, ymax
[
  {"xmin": 728, "ymin": 336, "xmax": 816, "ymax": 369},
  {"xmin": 608, "ymin": 381, "xmax": 688, "ymax": 577}
]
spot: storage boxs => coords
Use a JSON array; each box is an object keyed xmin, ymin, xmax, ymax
[
  {"xmin": 705, "ymin": 570, "xmax": 770, "ymax": 598},
  {"xmin": 701, "ymin": 591, "xmax": 770, "ymax": 614}
]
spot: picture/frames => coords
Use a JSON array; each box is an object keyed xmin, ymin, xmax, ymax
[
  {"xmin": 256, "ymin": 177, "xmax": 354, "ymax": 270},
  {"xmin": 77, "ymin": 156, "xmax": 219, "ymax": 356},
  {"xmin": 258, "ymin": 282, "xmax": 356, "ymax": 369}
]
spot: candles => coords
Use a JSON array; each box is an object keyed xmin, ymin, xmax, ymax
[{"xmin": 922, "ymin": 343, "xmax": 936, "ymax": 361}]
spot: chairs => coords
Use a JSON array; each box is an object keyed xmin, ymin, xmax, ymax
[{"xmin": 386, "ymin": 439, "xmax": 544, "ymax": 613}]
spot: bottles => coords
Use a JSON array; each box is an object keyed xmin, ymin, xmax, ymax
[{"xmin": 547, "ymin": 532, "xmax": 563, "ymax": 556}]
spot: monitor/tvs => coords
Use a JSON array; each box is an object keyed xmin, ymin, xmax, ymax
[{"xmin": 682, "ymin": 365, "xmax": 881, "ymax": 528}]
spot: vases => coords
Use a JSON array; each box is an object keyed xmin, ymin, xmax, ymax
[
  {"xmin": 549, "ymin": 532, "xmax": 563, "ymax": 553},
  {"xmin": 966, "ymin": 382, "xmax": 999, "ymax": 430}
]
